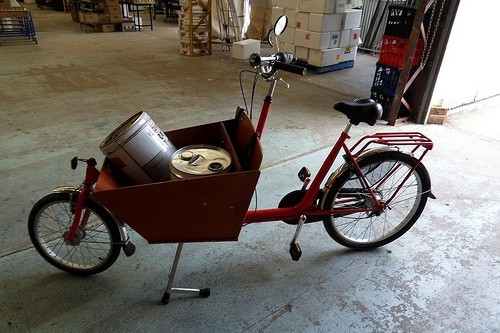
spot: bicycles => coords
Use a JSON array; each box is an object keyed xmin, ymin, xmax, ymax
[{"xmin": 28, "ymin": 14, "xmax": 436, "ymax": 305}]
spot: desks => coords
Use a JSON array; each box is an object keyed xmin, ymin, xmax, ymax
[{"xmin": 133, "ymin": 4, "xmax": 153, "ymax": 32}]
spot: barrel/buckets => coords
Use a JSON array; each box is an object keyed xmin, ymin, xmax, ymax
[
  {"xmin": 99, "ymin": 110, "xmax": 177, "ymax": 186},
  {"xmin": 169, "ymin": 144, "xmax": 232, "ymax": 180}
]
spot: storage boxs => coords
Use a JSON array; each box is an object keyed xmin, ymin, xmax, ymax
[
  {"xmin": 78, "ymin": 0, "xmax": 135, "ymax": 32},
  {"xmin": 229, "ymin": 0, "xmax": 432, "ymax": 122}
]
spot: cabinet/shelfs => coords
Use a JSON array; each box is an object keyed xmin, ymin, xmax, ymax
[
  {"xmin": 75, "ymin": 0, "xmax": 104, "ymax": 32},
  {"xmin": 178, "ymin": 0, "xmax": 211, "ymax": 58}
]
